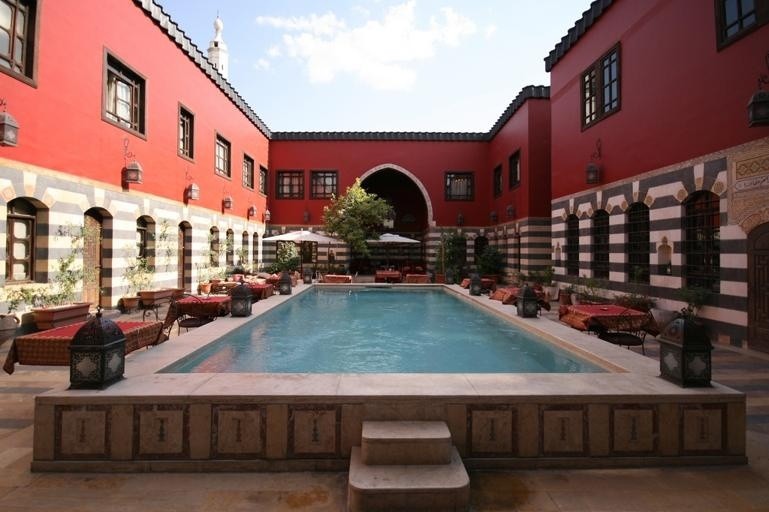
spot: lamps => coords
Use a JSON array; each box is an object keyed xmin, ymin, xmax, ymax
[
  {"xmin": 747, "ymin": 76, "xmax": 769, "ymax": 129},
  {"xmin": 506, "ymin": 204, "xmax": 513, "ymax": 219},
  {"xmin": 457, "ymin": 212, "xmax": 463, "ymax": 225},
  {"xmin": 490, "ymin": 212, "xmax": 496, "ymax": 222},
  {"xmin": 328, "ymin": 249, "xmax": 336, "ymax": 265},
  {"xmin": 67, "ymin": 305, "xmax": 127, "ymax": 388},
  {"xmin": 584, "ymin": 138, "xmax": 604, "ymax": 185},
  {"xmin": 656, "ymin": 316, "xmax": 714, "ymax": 388},
  {"xmin": 303, "ymin": 267, "xmax": 314, "ymax": 284},
  {"xmin": 0, "ymin": 97, "xmax": 270, "ymax": 222},
  {"xmin": 303, "ymin": 209, "xmax": 309, "ymax": 223}
]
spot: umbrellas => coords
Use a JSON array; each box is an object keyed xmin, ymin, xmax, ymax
[
  {"xmin": 260, "ymin": 228, "xmax": 348, "ymax": 278},
  {"xmin": 365, "ymin": 232, "xmax": 421, "ymax": 267}
]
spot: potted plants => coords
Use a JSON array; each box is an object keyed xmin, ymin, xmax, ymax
[
  {"xmin": 137, "ymin": 221, "xmax": 174, "ymax": 306},
  {"xmin": 29, "ymin": 226, "xmax": 91, "ymax": 330},
  {"xmin": 433, "ymin": 234, "xmax": 451, "ymax": 283},
  {"xmin": 120, "ymin": 259, "xmax": 143, "ymax": 311},
  {"xmin": 0, "ymin": 284, "xmax": 28, "ymax": 346},
  {"xmin": 211, "ymin": 237, "xmax": 228, "ymax": 290},
  {"xmin": 477, "ymin": 246, "xmax": 503, "ymax": 283}
]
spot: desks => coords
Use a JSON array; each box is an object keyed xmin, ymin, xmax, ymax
[
  {"xmin": 316, "ymin": 267, "xmax": 446, "ymax": 283},
  {"xmin": 2, "ymin": 272, "xmax": 300, "ymax": 376},
  {"xmin": 459, "ymin": 270, "xmax": 653, "ymax": 356}
]
